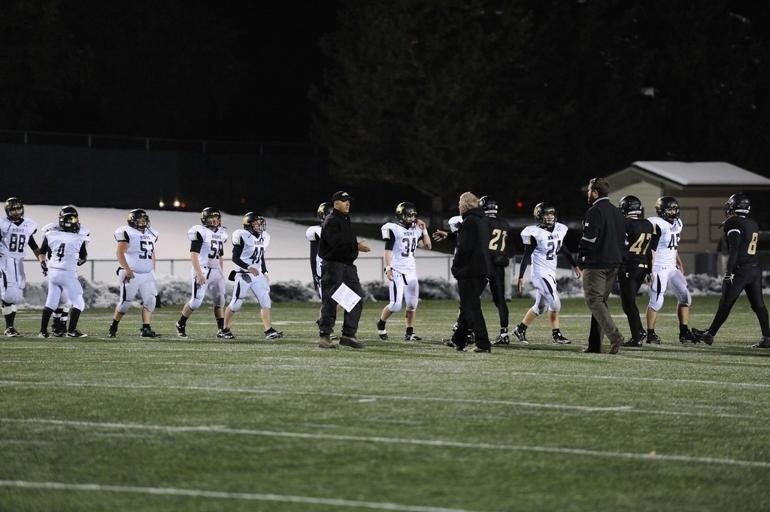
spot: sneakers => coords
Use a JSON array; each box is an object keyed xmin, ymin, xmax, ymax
[
  {"xmin": 582, "ymin": 348, "xmax": 602, "ymax": 354},
  {"xmin": 403, "ymin": 333, "xmax": 421, "ymax": 342},
  {"xmin": 52, "ymin": 321, "xmax": 63, "ymax": 337},
  {"xmin": 621, "ymin": 338, "xmax": 643, "ymax": 347},
  {"xmin": 140, "ymin": 328, "xmax": 161, "ymax": 339},
  {"xmin": 442, "ymin": 338, "xmax": 462, "ymax": 351},
  {"xmin": 265, "ymin": 331, "xmax": 283, "ymax": 340},
  {"xmin": 552, "ymin": 333, "xmax": 571, "ymax": 345},
  {"xmin": 470, "ymin": 347, "xmax": 491, "ymax": 354},
  {"xmin": 692, "ymin": 327, "xmax": 715, "ymax": 346},
  {"xmin": 339, "ymin": 333, "xmax": 365, "ymax": 348},
  {"xmin": 66, "ymin": 329, "xmax": 88, "ymax": 339},
  {"xmin": 376, "ymin": 321, "xmax": 389, "ymax": 343},
  {"xmin": 465, "ymin": 332, "xmax": 474, "ymax": 343},
  {"xmin": 635, "ymin": 328, "xmax": 648, "ymax": 345},
  {"xmin": 609, "ymin": 335, "xmax": 625, "ymax": 354},
  {"xmin": 490, "ymin": 335, "xmax": 510, "ymax": 345},
  {"xmin": 4, "ymin": 326, "xmax": 16, "ymax": 337},
  {"xmin": 646, "ymin": 331, "xmax": 661, "ymax": 344},
  {"xmin": 39, "ymin": 332, "xmax": 51, "ymax": 339},
  {"xmin": 679, "ymin": 329, "xmax": 695, "ymax": 343},
  {"xmin": 216, "ymin": 330, "xmax": 224, "ymax": 338},
  {"xmin": 753, "ymin": 336, "xmax": 770, "ymax": 348},
  {"xmin": 108, "ymin": 324, "xmax": 116, "ymax": 337},
  {"xmin": 175, "ymin": 322, "xmax": 188, "ymax": 339},
  {"xmin": 319, "ymin": 334, "xmax": 337, "ymax": 349},
  {"xmin": 14, "ymin": 328, "xmax": 23, "ymax": 337},
  {"xmin": 511, "ymin": 327, "xmax": 529, "ymax": 345},
  {"xmin": 221, "ymin": 331, "xmax": 234, "ymax": 340}
]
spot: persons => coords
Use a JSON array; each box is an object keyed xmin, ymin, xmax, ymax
[
  {"xmin": 223, "ymin": 211, "xmax": 285, "ymax": 339},
  {"xmin": 175, "ymin": 207, "xmax": 228, "ymax": 338},
  {"xmin": 38, "ymin": 213, "xmax": 88, "ymax": 338},
  {"xmin": 646, "ymin": 197, "xmax": 698, "ymax": 344},
  {"xmin": 690, "ymin": 193, "xmax": 770, "ymax": 348},
  {"xmin": 42, "ymin": 204, "xmax": 91, "ymax": 337},
  {"xmin": 376, "ymin": 202, "xmax": 432, "ymax": 342},
  {"xmin": 576, "ymin": 178, "xmax": 624, "ymax": 354},
  {"xmin": 466, "ymin": 196, "xmax": 511, "ymax": 345},
  {"xmin": 108, "ymin": 209, "xmax": 160, "ymax": 338},
  {"xmin": 432, "ymin": 191, "xmax": 494, "ymax": 353},
  {"xmin": 617, "ymin": 195, "xmax": 654, "ymax": 347},
  {"xmin": 511, "ymin": 200, "xmax": 582, "ymax": 346},
  {"xmin": 306, "ymin": 202, "xmax": 344, "ymax": 339},
  {"xmin": 316, "ymin": 190, "xmax": 371, "ymax": 348},
  {"xmin": 0, "ymin": 197, "xmax": 40, "ymax": 337}
]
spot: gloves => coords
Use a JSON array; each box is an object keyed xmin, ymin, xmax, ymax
[
  {"xmin": 724, "ymin": 272, "xmax": 735, "ymax": 286},
  {"xmin": 647, "ymin": 273, "xmax": 654, "ymax": 285}
]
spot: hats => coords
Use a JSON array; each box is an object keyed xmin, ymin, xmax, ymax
[{"xmin": 333, "ymin": 190, "xmax": 354, "ymax": 202}]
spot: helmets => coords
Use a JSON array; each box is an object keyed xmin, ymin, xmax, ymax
[
  {"xmin": 5, "ymin": 198, "xmax": 24, "ymax": 219},
  {"xmin": 58, "ymin": 206, "xmax": 78, "ymax": 218},
  {"xmin": 242, "ymin": 211, "xmax": 266, "ymax": 235},
  {"xmin": 655, "ymin": 196, "xmax": 681, "ymax": 221},
  {"xmin": 620, "ymin": 196, "xmax": 645, "ymax": 218},
  {"xmin": 60, "ymin": 214, "xmax": 81, "ymax": 232},
  {"xmin": 200, "ymin": 207, "xmax": 223, "ymax": 229},
  {"xmin": 127, "ymin": 209, "xmax": 151, "ymax": 231},
  {"xmin": 395, "ymin": 202, "xmax": 418, "ymax": 228},
  {"xmin": 480, "ymin": 196, "xmax": 500, "ymax": 215},
  {"xmin": 724, "ymin": 195, "xmax": 752, "ymax": 215},
  {"xmin": 534, "ymin": 202, "xmax": 556, "ymax": 229},
  {"xmin": 316, "ymin": 203, "xmax": 333, "ymax": 224}
]
